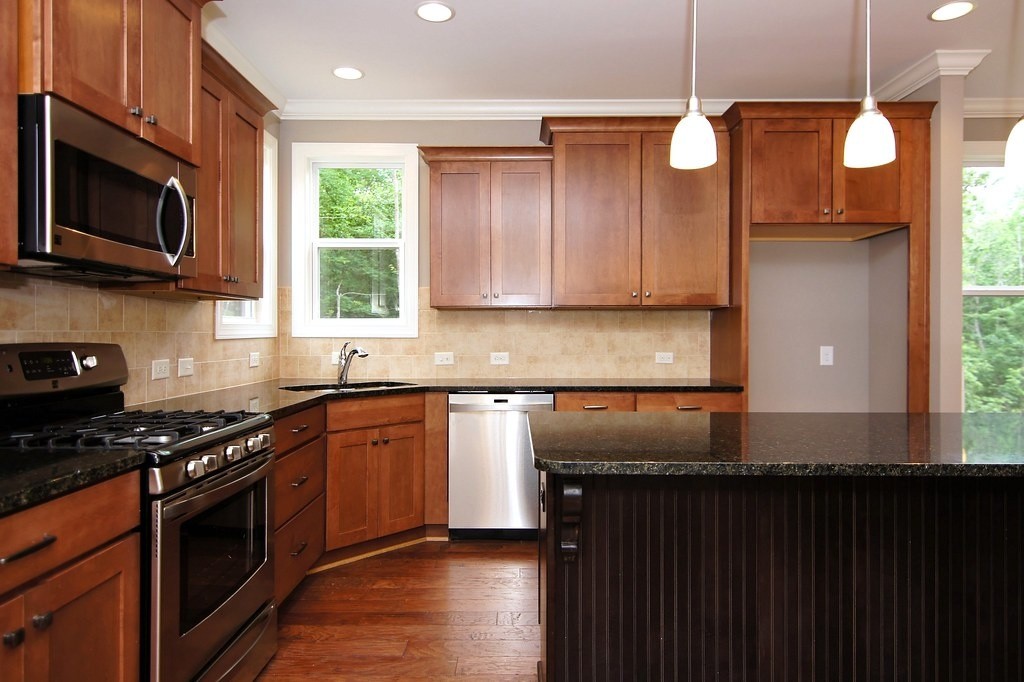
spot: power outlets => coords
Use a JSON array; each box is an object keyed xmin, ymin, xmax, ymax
[
  {"xmin": 656, "ymin": 352, "xmax": 674, "ymax": 363},
  {"xmin": 435, "ymin": 352, "xmax": 454, "ymax": 365},
  {"xmin": 151, "ymin": 352, "xmax": 260, "ymax": 380},
  {"xmin": 491, "ymin": 353, "xmax": 509, "ymax": 364}
]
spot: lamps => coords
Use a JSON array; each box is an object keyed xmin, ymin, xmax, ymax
[
  {"xmin": 670, "ymin": 0, "xmax": 717, "ymax": 170},
  {"xmin": 843, "ymin": 0, "xmax": 897, "ymax": 168},
  {"xmin": 1005, "ymin": 117, "xmax": 1024, "ymax": 190}
]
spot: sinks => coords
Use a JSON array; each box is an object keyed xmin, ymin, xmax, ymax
[{"xmin": 297, "ymin": 386, "xmax": 401, "ymax": 393}]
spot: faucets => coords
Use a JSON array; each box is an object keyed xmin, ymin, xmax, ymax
[{"xmin": 337, "ymin": 341, "xmax": 370, "ymax": 385}]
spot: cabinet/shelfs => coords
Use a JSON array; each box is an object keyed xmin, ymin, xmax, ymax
[
  {"xmin": 273, "ymin": 394, "xmax": 423, "ymax": 605},
  {"xmin": 0, "ymin": 471, "xmax": 141, "ymax": 682},
  {"xmin": 0, "ymin": 0, "xmax": 263, "ymax": 298},
  {"xmin": 415, "ymin": 118, "xmax": 915, "ymax": 307},
  {"xmin": 555, "ymin": 392, "xmax": 741, "ymax": 413}
]
